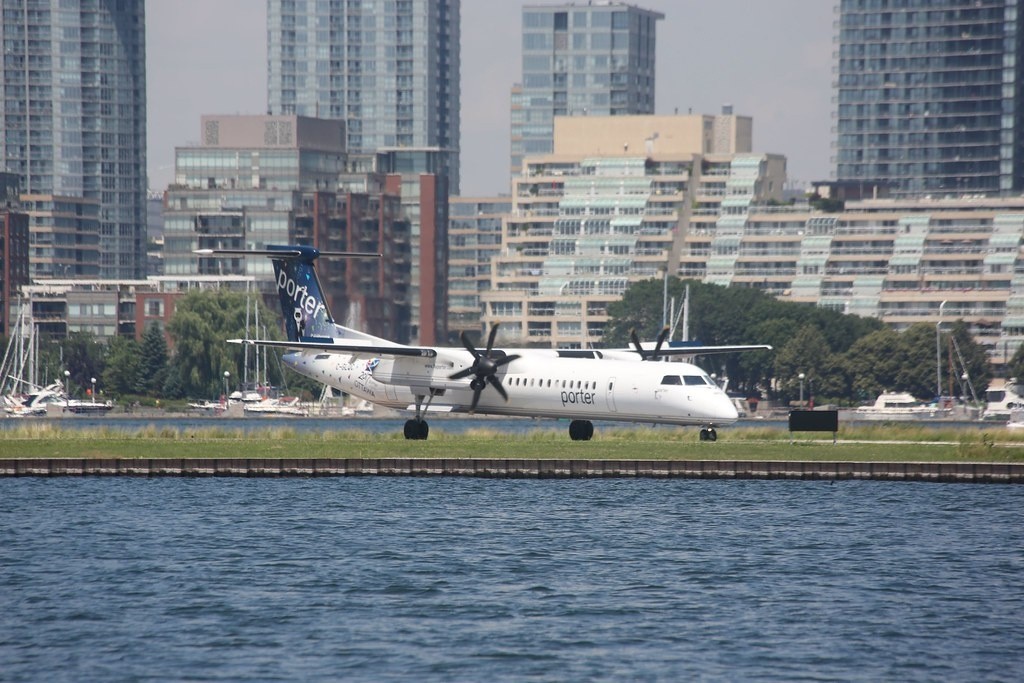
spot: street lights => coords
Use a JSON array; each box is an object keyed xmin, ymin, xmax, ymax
[
  {"xmin": 224, "ymin": 371, "xmax": 230, "ymax": 410},
  {"xmin": 64, "ymin": 371, "xmax": 70, "ymax": 412},
  {"xmin": 91, "ymin": 377, "xmax": 96, "ymax": 404},
  {"xmin": 961, "ymin": 373, "xmax": 968, "ymax": 416},
  {"xmin": 798, "ymin": 372, "xmax": 805, "ymax": 410}
]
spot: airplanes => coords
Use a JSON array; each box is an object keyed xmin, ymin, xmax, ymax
[{"xmin": 192, "ymin": 244, "xmax": 773, "ymax": 441}]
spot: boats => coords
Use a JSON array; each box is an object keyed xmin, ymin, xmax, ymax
[{"xmin": 856, "ymin": 390, "xmax": 953, "ymax": 415}]
